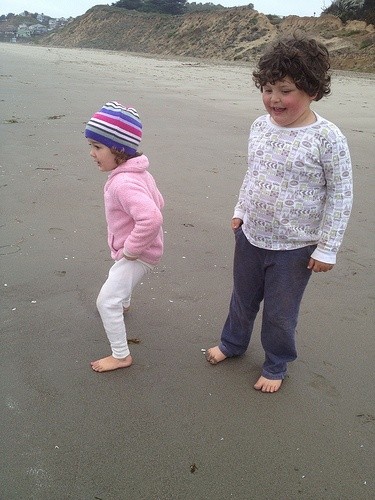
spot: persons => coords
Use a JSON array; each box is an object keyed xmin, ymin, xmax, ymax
[
  {"xmin": 206, "ymin": 39, "xmax": 353, "ymax": 392},
  {"xmin": 84, "ymin": 101, "xmax": 165, "ymax": 372}
]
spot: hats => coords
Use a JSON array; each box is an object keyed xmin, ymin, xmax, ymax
[{"xmin": 84, "ymin": 100, "xmax": 143, "ymax": 156}]
